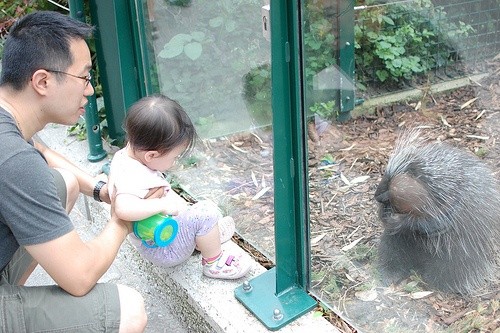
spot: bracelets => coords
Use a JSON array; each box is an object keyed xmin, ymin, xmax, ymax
[{"xmin": 93, "ymin": 180, "xmax": 107, "ymax": 203}]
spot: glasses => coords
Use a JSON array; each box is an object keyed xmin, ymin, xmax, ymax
[{"xmin": 30, "ymin": 69, "xmax": 91, "ymax": 86}]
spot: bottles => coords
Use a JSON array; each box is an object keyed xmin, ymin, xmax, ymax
[{"xmin": 128, "ymin": 212, "xmax": 178, "ymax": 250}]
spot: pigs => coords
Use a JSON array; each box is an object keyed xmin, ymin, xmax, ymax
[{"xmin": 374, "ymin": 119, "xmax": 500, "ymax": 303}]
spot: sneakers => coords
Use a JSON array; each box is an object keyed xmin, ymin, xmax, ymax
[
  {"xmin": 201, "ymin": 254, "xmax": 251, "ymax": 280},
  {"xmin": 221, "ymin": 215, "xmax": 235, "ymax": 244}
]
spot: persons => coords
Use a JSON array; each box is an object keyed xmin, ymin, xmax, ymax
[
  {"xmin": 106, "ymin": 92, "xmax": 249, "ymax": 280},
  {"xmin": 0, "ymin": 9, "xmax": 168, "ymax": 333}
]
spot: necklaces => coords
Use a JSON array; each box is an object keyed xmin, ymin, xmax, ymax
[{"xmin": 0, "ymin": 103, "xmax": 22, "ymax": 135}]
